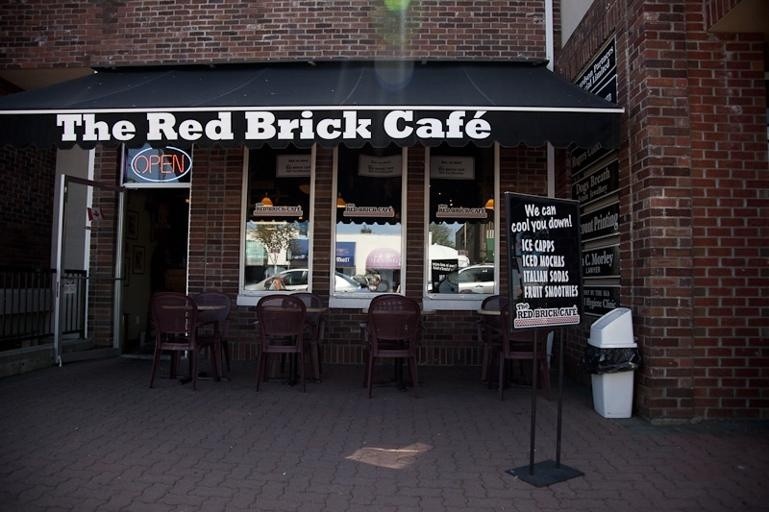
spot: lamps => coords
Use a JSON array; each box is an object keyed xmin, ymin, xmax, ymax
[
  {"xmin": 337, "ymin": 193, "xmax": 346, "ymax": 208},
  {"xmin": 259, "ymin": 191, "xmax": 273, "ymax": 205},
  {"xmin": 485, "ymin": 192, "xmax": 494, "ymax": 210}
]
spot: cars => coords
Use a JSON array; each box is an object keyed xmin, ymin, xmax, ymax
[
  {"xmin": 245, "ymin": 268, "xmax": 364, "ymax": 291},
  {"xmin": 458, "ymin": 261, "xmax": 494, "ymax": 294}
]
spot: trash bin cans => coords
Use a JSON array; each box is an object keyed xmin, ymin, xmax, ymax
[{"xmin": 587, "ymin": 307, "xmax": 638, "ymax": 419}]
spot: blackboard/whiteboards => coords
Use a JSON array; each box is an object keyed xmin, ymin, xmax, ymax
[{"xmin": 505, "ymin": 190, "xmax": 586, "ymax": 333}]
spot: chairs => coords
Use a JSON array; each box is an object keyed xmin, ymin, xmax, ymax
[
  {"xmin": 253, "ymin": 292, "xmax": 329, "ymax": 394},
  {"xmin": 477, "ymin": 293, "xmax": 559, "ymax": 400},
  {"xmin": 141, "ymin": 290, "xmax": 234, "ymax": 393},
  {"xmin": 359, "ymin": 294, "xmax": 425, "ymax": 399}
]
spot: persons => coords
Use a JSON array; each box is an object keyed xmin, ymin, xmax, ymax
[{"xmin": 371, "ymin": 274, "xmax": 381, "ymax": 293}]
